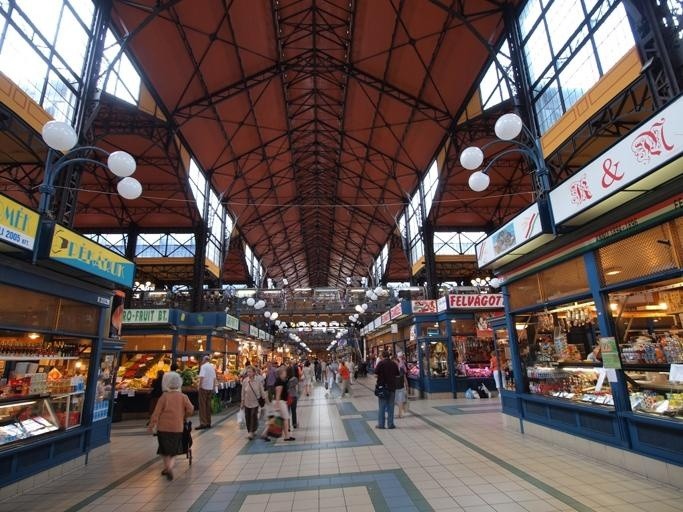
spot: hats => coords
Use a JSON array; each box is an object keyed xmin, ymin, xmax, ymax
[{"xmin": 162, "ymin": 371, "xmax": 184, "ymax": 392}]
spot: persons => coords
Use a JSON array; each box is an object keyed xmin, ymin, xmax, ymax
[
  {"xmin": 489, "ymin": 351, "xmax": 507, "ymax": 397},
  {"xmin": 144, "ymin": 371, "xmax": 196, "ymax": 481},
  {"xmin": 132, "ymin": 349, "xmax": 411, "ymax": 443}
]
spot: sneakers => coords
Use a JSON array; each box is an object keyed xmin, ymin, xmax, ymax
[
  {"xmin": 375, "ymin": 425, "xmax": 395, "ymax": 429},
  {"xmin": 161, "ymin": 469, "xmax": 173, "ymax": 481},
  {"xmin": 261, "ymin": 435, "xmax": 270, "ymax": 441},
  {"xmin": 284, "ymin": 437, "xmax": 295, "ymax": 441},
  {"xmin": 247, "ymin": 431, "xmax": 257, "ymax": 440}
]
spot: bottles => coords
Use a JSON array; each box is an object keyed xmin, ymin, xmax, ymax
[
  {"xmin": 525, "ymin": 366, "xmax": 574, "ymax": 395},
  {"xmin": 539, "ymin": 341, "xmax": 586, "ymax": 364},
  {"xmin": 562, "ymin": 372, "xmax": 596, "ymax": 396},
  {"xmin": 0, "ymin": 340, "xmax": 80, "ymax": 358},
  {"xmin": 619, "ymin": 330, "xmax": 683, "ymax": 365},
  {"xmin": 0, "ymin": 370, "xmax": 85, "ymax": 398},
  {"xmin": 563, "ymin": 307, "xmax": 593, "ymax": 329}
]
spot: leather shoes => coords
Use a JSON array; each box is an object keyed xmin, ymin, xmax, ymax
[{"xmin": 195, "ymin": 424, "xmax": 210, "ymax": 430}]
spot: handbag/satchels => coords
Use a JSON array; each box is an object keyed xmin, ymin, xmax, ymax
[
  {"xmin": 374, "ymin": 383, "xmax": 386, "ymax": 399},
  {"xmin": 324, "ymin": 382, "xmax": 328, "ymax": 389},
  {"xmin": 465, "ymin": 383, "xmax": 492, "ymax": 399},
  {"xmin": 267, "ymin": 415, "xmax": 284, "ymax": 438},
  {"xmin": 301, "ymin": 373, "xmax": 305, "ymax": 380},
  {"xmin": 257, "ymin": 395, "xmax": 265, "ymax": 407}
]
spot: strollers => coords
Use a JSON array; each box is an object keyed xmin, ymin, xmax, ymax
[{"xmin": 146, "ymin": 419, "xmax": 194, "ymax": 468}]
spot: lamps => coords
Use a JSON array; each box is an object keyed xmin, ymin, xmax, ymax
[
  {"xmin": 38, "ymin": 120, "xmax": 142, "ymax": 213},
  {"xmin": 458, "ymin": 112, "xmax": 549, "ymax": 193}
]
